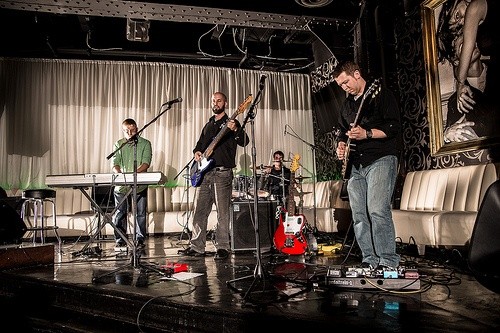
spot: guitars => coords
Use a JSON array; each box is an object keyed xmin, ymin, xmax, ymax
[
  {"xmin": 189, "ymin": 94, "xmax": 253, "ymax": 187},
  {"xmin": 273, "ymin": 154, "xmax": 308, "ymax": 256},
  {"xmin": 341, "ymin": 77, "xmax": 383, "ymax": 180}
]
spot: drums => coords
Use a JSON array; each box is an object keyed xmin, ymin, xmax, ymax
[
  {"xmin": 229, "ymin": 173, "xmax": 252, "ymax": 197},
  {"xmin": 269, "ymin": 174, "xmax": 291, "ymax": 197},
  {"xmin": 247, "ymin": 174, "xmax": 273, "ymax": 198}
]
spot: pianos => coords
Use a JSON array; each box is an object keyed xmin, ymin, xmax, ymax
[{"xmin": 44, "ymin": 171, "xmax": 167, "ymax": 256}]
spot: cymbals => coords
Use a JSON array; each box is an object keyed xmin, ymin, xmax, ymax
[
  {"xmin": 295, "ymin": 176, "xmax": 312, "ymax": 179},
  {"xmin": 270, "ymin": 159, "xmax": 291, "ymax": 163},
  {"xmin": 253, "ymin": 165, "xmax": 272, "ymax": 170},
  {"xmin": 294, "ymin": 192, "xmax": 312, "ymax": 196}
]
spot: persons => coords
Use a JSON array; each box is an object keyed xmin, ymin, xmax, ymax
[
  {"xmin": 113, "ymin": 118, "xmax": 152, "ymax": 251},
  {"xmin": 436, "ymin": 0, "xmax": 500, "ymax": 143},
  {"xmin": 333, "ymin": 60, "xmax": 402, "ymax": 271},
  {"xmin": 177, "ymin": 92, "xmax": 249, "ymax": 259},
  {"xmin": 261, "ymin": 151, "xmax": 291, "ymax": 228}
]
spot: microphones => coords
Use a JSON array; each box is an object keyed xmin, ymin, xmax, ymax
[
  {"xmin": 162, "ymin": 97, "xmax": 182, "ymax": 106},
  {"xmin": 288, "ymin": 153, "xmax": 290, "ymax": 161},
  {"xmin": 284, "ymin": 124, "xmax": 287, "ymax": 135},
  {"xmin": 263, "ymin": 75, "xmax": 266, "ymax": 88}
]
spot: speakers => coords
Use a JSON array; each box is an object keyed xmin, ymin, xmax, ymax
[
  {"xmin": 227, "ymin": 200, "xmax": 274, "ymax": 251},
  {"xmin": 468, "ymin": 179, "xmax": 500, "ymax": 296}
]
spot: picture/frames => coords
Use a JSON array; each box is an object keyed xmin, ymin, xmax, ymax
[{"xmin": 420, "ymin": 0, "xmax": 500, "ymax": 158}]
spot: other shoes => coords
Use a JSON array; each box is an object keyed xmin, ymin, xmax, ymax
[
  {"xmin": 137, "ymin": 248, "xmax": 146, "ymax": 256},
  {"xmin": 355, "ymin": 261, "xmax": 374, "ymax": 272},
  {"xmin": 213, "ymin": 249, "xmax": 229, "ymax": 259},
  {"xmin": 177, "ymin": 247, "xmax": 206, "ymax": 257},
  {"xmin": 114, "ymin": 242, "xmax": 128, "ymax": 251},
  {"xmin": 373, "ymin": 265, "xmax": 398, "ymax": 276}
]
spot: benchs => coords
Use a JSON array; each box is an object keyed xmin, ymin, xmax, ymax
[
  {"xmin": 294, "ymin": 180, "xmax": 348, "ymax": 245},
  {"xmin": 391, "ymin": 163, "xmax": 497, "ymax": 257}
]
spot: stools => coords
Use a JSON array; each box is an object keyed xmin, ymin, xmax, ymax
[{"xmin": 21, "ymin": 188, "xmax": 63, "ymax": 244}]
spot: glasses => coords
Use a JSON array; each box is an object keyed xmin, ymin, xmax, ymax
[{"xmin": 122, "ymin": 128, "xmax": 136, "ymax": 134}]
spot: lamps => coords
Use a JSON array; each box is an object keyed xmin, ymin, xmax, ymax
[{"xmin": 125, "ymin": 18, "xmax": 151, "ymax": 43}]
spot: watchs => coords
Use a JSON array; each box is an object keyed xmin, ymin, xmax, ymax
[{"xmin": 366, "ymin": 128, "xmax": 372, "ymax": 139}]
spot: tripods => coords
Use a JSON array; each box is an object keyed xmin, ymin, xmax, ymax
[
  {"xmin": 92, "ymin": 105, "xmax": 171, "ymax": 281},
  {"xmin": 224, "ymin": 85, "xmax": 312, "ymax": 303}
]
